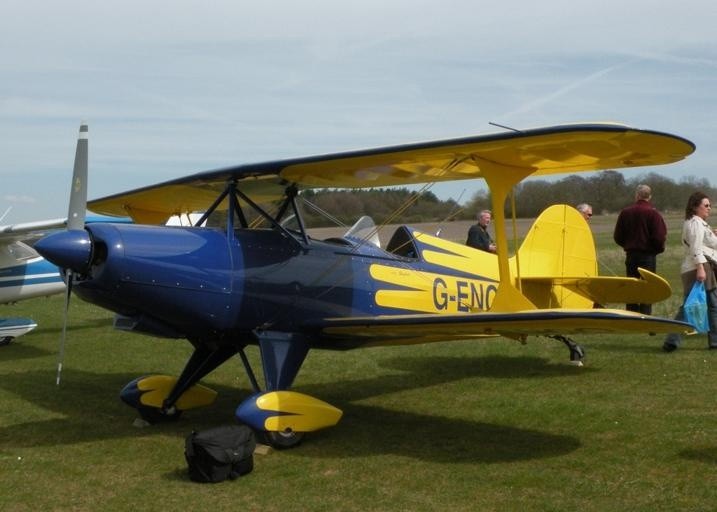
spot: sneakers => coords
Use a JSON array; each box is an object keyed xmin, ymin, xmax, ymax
[{"xmin": 662, "ymin": 339, "xmax": 678, "ymax": 354}]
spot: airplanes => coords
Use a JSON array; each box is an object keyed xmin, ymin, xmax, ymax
[
  {"xmin": 35, "ymin": 113, "xmax": 702, "ymax": 457},
  {"xmin": 0, "ymin": 216, "xmax": 134, "ymax": 347}
]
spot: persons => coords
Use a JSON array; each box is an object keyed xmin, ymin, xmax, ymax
[
  {"xmin": 464, "ymin": 209, "xmax": 496, "ymax": 255},
  {"xmin": 576, "ymin": 202, "xmax": 593, "ymax": 224},
  {"xmin": 612, "ymin": 183, "xmax": 666, "ymax": 337},
  {"xmin": 661, "ymin": 190, "xmax": 716, "ymax": 353}
]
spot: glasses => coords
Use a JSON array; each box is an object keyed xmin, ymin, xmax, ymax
[{"xmin": 704, "ymin": 203, "xmax": 711, "ymax": 208}]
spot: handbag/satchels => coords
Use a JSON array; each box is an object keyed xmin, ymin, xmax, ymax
[{"xmin": 184, "ymin": 425, "xmax": 257, "ymax": 483}]
